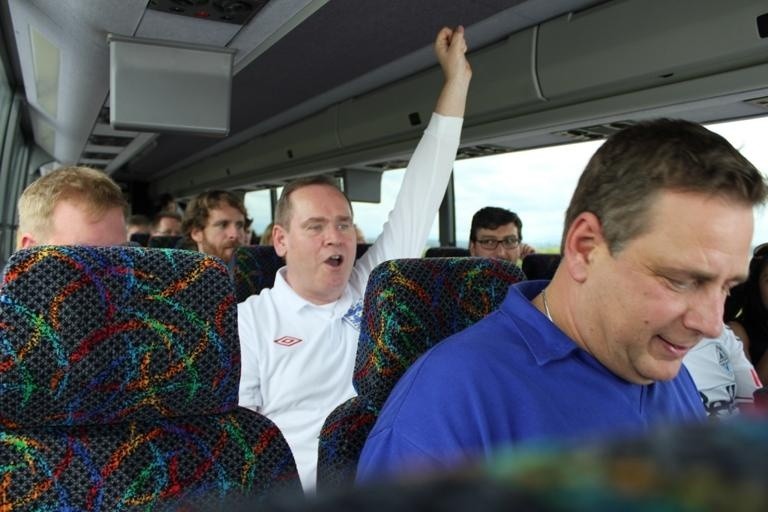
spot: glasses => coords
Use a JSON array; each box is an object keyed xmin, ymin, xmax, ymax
[
  {"xmin": 152, "ymin": 228, "xmax": 185, "ymax": 237},
  {"xmin": 473, "ymin": 235, "xmax": 523, "ymax": 251}
]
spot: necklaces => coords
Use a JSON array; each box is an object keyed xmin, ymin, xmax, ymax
[{"xmin": 540, "ymin": 286, "xmax": 556, "ymax": 325}]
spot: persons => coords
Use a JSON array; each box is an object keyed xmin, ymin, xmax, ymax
[
  {"xmin": 16, "ymin": 166, "xmax": 127, "ymax": 250},
  {"xmin": 470, "ymin": 206, "xmax": 536, "ymax": 268},
  {"xmin": 354, "ymin": 117, "xmax": 767, "ymax": 512},
  {"xmin": 723, "ymin": 243, "xmax": 767, "ymax": 417},
  {"xmin": 683, "ymin": 325, "xmax": 763, "ymax": 417},
  {"xmin": 180, "ymin": 189, "xmax": 247, "ymax": 264},
  {"xmin": 163, "ymin": 200, "xmax": 184, "ymax": 218},
  {"xmin": 154, "ymin": 215, "xmax": 184, "ymax": 237},
  {"xmin": 128, "ymin": 215, "xmax": 151, "ymax": 241},
  {"xmin": 238, "ymin": 23, "xmax": 472, "ymax": 495}
]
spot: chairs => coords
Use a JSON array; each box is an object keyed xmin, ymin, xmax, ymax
[
  {"xmin": 229, "ymin": 245, "xmax": 283, "ymax": 306},
  {"xmin": 0, "ymin": 244, "xmax": 306, "ymax": 512},
  {"xmin": 316, "ymin": 255, "xmax": 529, "ymax": 511}
]
